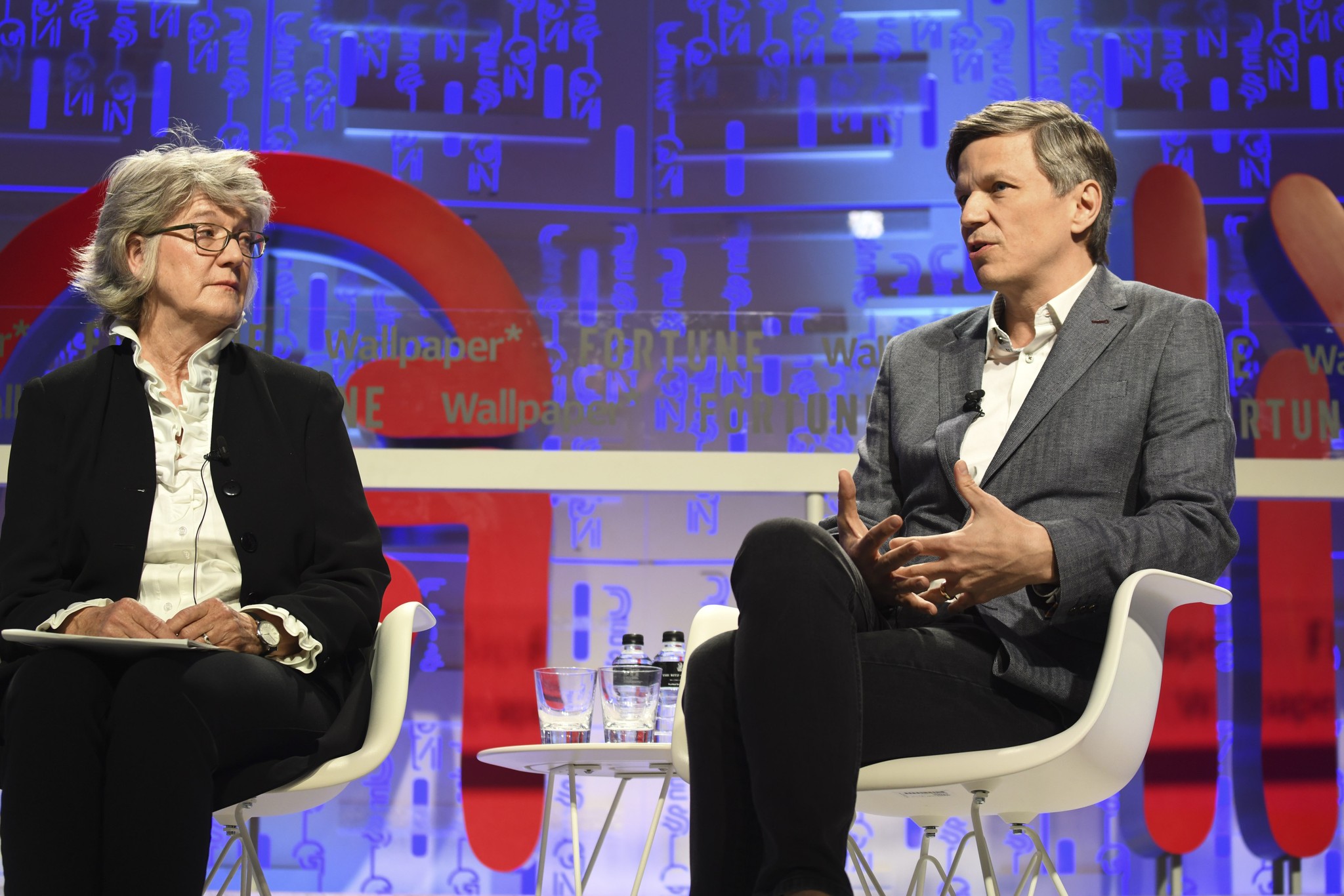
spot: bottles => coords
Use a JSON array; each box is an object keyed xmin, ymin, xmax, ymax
[
  {"xmin": 652, "ymin": 631, "xmax": 688, "ymax": 743},
  {"xmin": 613, "ymin": 634, "xmax": 652, "ymax": 716}
]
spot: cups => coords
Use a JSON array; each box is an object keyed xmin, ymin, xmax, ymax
[
  {"xmin": 599, "ymin": 665, "xmax": 663, "ymax": 743},
  {"xmin": 534, "ymin": 667, "xmax": 599, "ymax": 744}
]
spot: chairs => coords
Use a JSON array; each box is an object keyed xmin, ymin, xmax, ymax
[
  {"xmin": 202, "ymin": 601, "xmax": 437, "ymax": 896},
  {"xmin": 673, "ymin": 568, "xmax": 1232, "ymax": 896}
]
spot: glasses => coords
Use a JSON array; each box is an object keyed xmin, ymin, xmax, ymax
[{"xmin": 147, "ymin": 223, "xmax": 269, "ymax": 259}]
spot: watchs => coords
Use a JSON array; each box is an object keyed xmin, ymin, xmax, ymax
[{"xmin": 256, "ymin": 620, "xmax": 280, "ymax": 656}]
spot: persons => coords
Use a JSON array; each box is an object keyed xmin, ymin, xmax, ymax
[
  {"xmin": 0, "ymin": 146, "xmax": 391, "ymax": 896},
  {"xmin": 681, "ymin": 99, "xmax": 1241, "ymax": 896}
]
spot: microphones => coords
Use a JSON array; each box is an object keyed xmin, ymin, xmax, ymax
[
  {"xmin": 216, "ymin": 435, "xmax": 230, "ymax": 463},
  {"xmin": 964, "ymin": 389, "xmax": 985, "ymax": 401}
]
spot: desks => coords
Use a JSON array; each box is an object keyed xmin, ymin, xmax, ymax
[{"xmin": 476, "ymin": 742, "xmax": 886, "ymax": 896}]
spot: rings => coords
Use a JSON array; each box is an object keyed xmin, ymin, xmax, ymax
[{"xmin": 202, "ymin": 634, "xmax": 212, "ymax": 645}]
